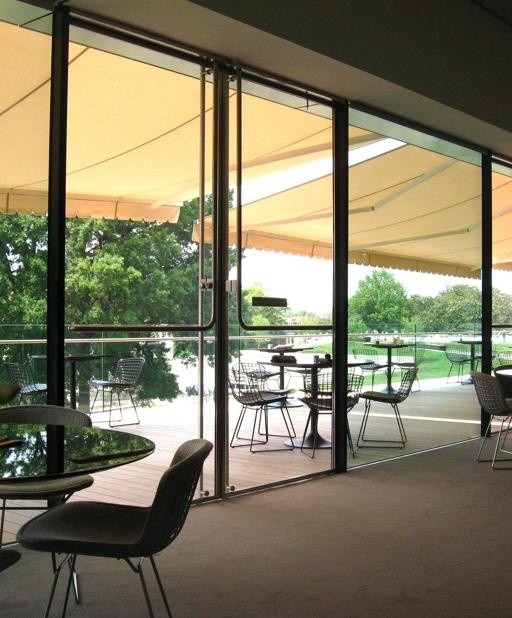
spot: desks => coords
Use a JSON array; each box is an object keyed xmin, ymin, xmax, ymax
[{"xmin": 0, "ymin": 426, "xmax": 154, "ymax": 601}]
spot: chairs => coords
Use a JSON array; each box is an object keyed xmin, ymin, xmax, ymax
[
  {"xmin": 229, "ymin": 342, "xmax": 511, "ymax": 471},
  {"xmin": 7, "ymin": 350, "xmax": 147, "ymax": 426},
  {"xmin": 16, "ymin": 438, "xmax": 214, "ymax": 617},
  {"xmin": 3, "ymin": 405, "xmax": 91, "ymax": 606}
]
